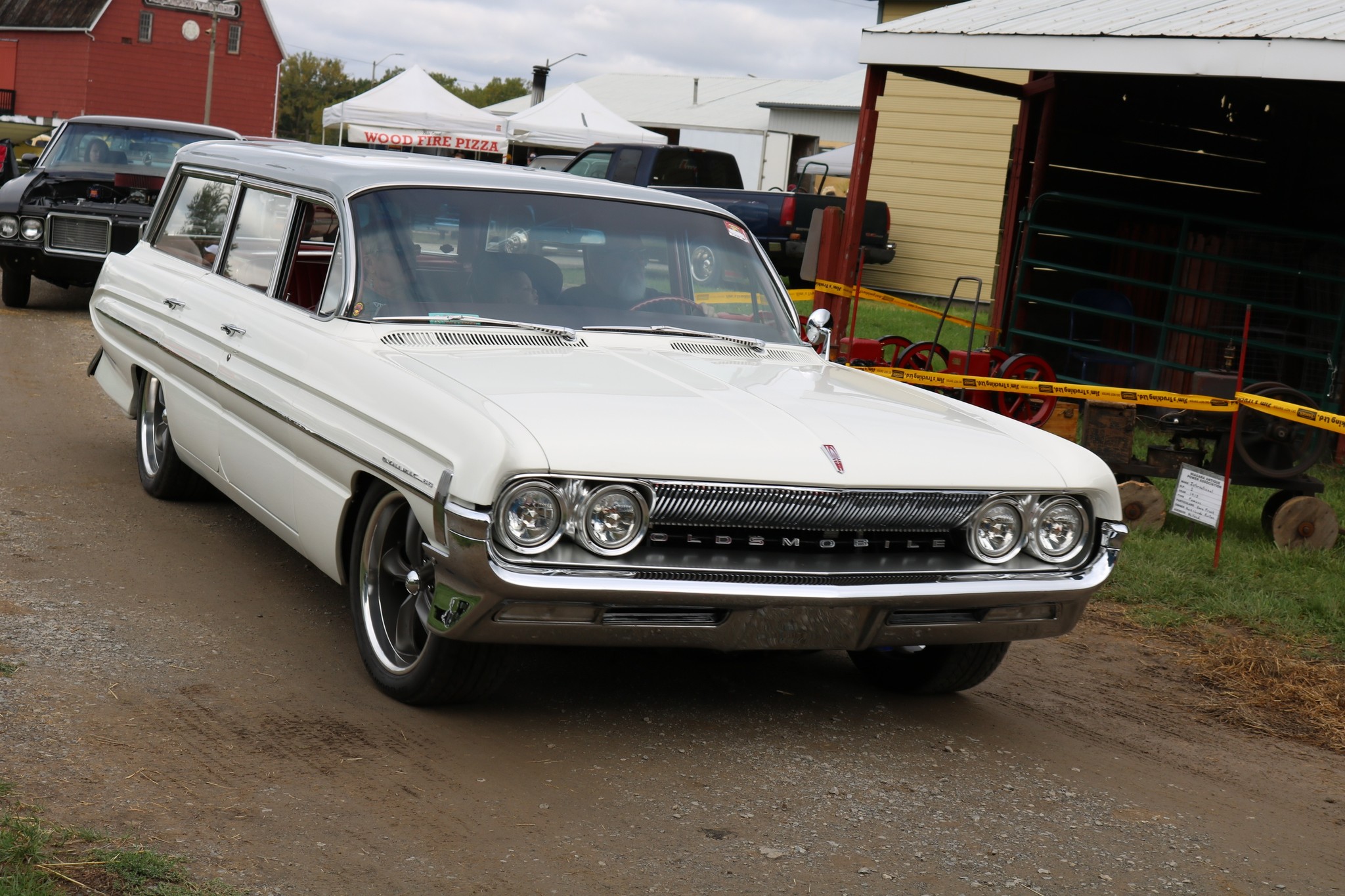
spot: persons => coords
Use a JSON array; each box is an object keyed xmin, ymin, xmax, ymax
[
  {"xmin": 486, "ymin": 265, "xmax": 541, "ymax": 308},
  {"xmin": 83, "ymin": 139, "xmax": 110, "ymax": 164},
  {"xmin": 554, "ymin": 219, "xmax": 719, "ymax": 321}
]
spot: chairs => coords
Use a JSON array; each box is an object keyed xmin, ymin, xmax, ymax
[
  {"xmin": 472, "ymin": 249, "xmax": 566, "ymax": 308},
  {"xmin": 106, "ymin": 150, "xmax": 128, "ymax": 166}
]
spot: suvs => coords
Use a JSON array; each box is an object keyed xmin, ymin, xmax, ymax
[{"xmin": 0, "ymin": 115, "xmax": 339, "ymax": 308}]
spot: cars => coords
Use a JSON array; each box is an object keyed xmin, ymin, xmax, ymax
[
  {"xmin": 86, "ymin": 140, "xmax": 1131, "ymax": 705},
  {"xmin": 434, "ymin": 156, "xmax": 609, "ymax": 236}
]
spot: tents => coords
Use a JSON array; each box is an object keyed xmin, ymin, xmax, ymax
[
  {"xmin": 504, "ymin": 82, "xmax": 668, "ymax": 169},
  {"xmin": 796, "ymin": 143, "xmax": 856, "ymax": 178},
  {"xmin": 321, "ymin": 63, "xmax": 509, "ymax": 166}
]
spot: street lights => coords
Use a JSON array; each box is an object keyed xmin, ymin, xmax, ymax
[
  {"xmin": 272, "ymin": 60, "xmax": 287, "ymax": 138},
  {"xmin": 372, "ymin": 54, "xmax": 403, "ymax": 89},
  {"xmin": 542, "ymin": 53, "xmax": 588, "ymax": 98}
]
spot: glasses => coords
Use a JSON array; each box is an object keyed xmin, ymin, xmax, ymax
[{"xmin": 606, "ymin": 247, "xmax": 650, "ymax": 268}]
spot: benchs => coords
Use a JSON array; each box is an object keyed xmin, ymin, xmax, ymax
[{"xmin": 242, "ymin": 251, "xmax": 457, "ymax": 304}]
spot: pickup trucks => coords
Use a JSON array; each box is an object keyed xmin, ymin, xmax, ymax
[{"xmin": 498, "ymin": 143, "xmax": 895, "ymax": 289}]
